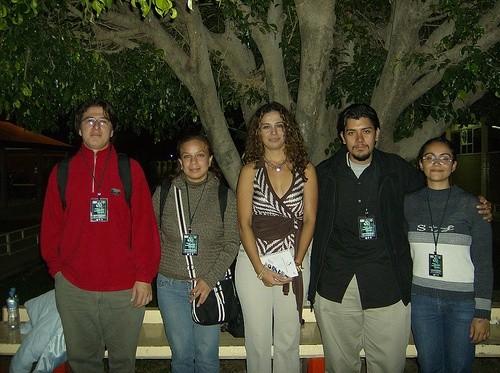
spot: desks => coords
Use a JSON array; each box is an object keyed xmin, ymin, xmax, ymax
[{"xmin": 0, "ymin": 306, "xmax": 500, "ymax": 373}]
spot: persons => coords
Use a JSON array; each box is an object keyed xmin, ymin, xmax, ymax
[
  {"xmin": 40, "ymin": 97, "xmax": 161, "ymax": 373},
  {"xmin": 151, "ymin": 133, "xmax": 243, "ymax": 373},
  {"xmin": 234, "ymin": 101, "xmax": 318, "ymax": 373},
  {"xmin": 306, "ymin": 102, "xmax": 493, "ymax": 373},
  {"xmin": 403, "ymin": 137, "xmax": 493, "ymax": 373}
]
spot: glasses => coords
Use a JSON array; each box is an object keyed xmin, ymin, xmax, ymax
[
  {"xmin": 421, "ymin": 153, "xmax": 453, "ymax": 166},
  {"xmin": 80, "ymin": 117, "xmax": 112, "ymax": 131}
]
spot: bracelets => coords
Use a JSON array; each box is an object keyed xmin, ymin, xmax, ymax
[
  {"xmin": 295, "ymin": 262, "xmax": 304, "ymax": 272},
  {"xmin": 257, "ymin": 266, "xmax": 269, "ymax": 280}
]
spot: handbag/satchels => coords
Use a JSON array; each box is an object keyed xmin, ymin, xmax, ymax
[{"xmin": 192, "ymin": 278, "xmax": 236, "ymax": 325}]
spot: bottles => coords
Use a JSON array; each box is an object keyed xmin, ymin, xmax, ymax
[{"xmin": 6, "ymin": 288, "xmax": 20, "ymax": 330}]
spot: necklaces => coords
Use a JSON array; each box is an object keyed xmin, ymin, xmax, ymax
[{"xmin": 263, "ymin": 156, "xmax": 287, "ymax": 172}]
